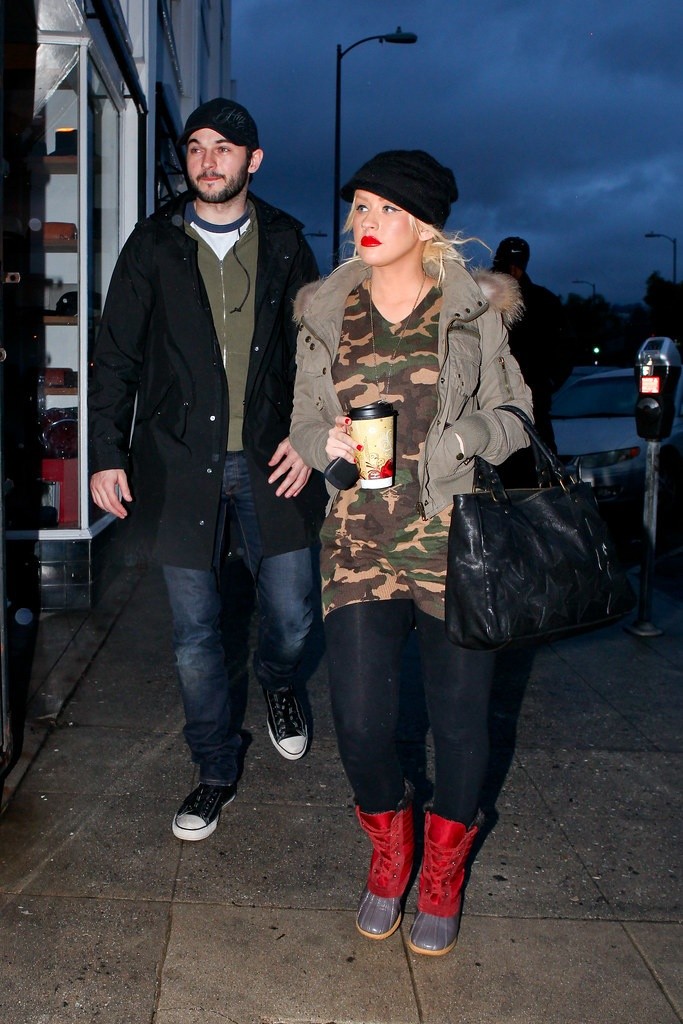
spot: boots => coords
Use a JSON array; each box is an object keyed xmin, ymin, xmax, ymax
[
  {"xmin": 408, "ymin": 797, "xmax": 485, "ymax": 956},
  {"xmin": 353, "ymin": 782, "xmax": 415, "ymax": 939}
]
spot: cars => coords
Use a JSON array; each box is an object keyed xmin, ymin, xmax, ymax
[{"xmin": 549, "ymin": 368, "xmax": 682, "ymax": 520}]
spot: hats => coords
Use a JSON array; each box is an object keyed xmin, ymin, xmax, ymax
[
  {"xmin": 177, "ymin": 97, "xmax": 259, "ymax": 150},
  {"xmin": 492, "ymin": 236, "xmax": 530, "ymax": 267},
  {"xmin": 340, "ymin": 149, "xmax": 459, "ymax": 233}
]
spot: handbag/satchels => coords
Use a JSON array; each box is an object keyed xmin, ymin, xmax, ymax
[{"xmin": 444, "ymin": 405, "xmax": 637, "ymax": 651}]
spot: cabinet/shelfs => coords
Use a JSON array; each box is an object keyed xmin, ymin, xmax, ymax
[{"xmin": 31, "ymin": 67, "xmax": 102, "ymax": 529}]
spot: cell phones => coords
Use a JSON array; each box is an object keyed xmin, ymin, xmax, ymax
[{"xmin": 325, "ymin": 456, "xmax": 360, "ymax": 491}]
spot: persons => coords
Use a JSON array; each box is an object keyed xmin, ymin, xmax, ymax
[
  {"xmin": 489, "ymin": 237, "xmax": 565, "ymax": 489},
  {"xmin": 290, "ymin": 151, "xmax": 534, "ymax": 956},
  {"xmin": 87, "ymin": 97, "xmax": 320, "ymax": 842}
]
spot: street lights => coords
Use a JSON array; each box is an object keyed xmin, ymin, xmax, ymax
[
  {"xmin": 332, "ymin": 23, "xmax": 418, "ymax": 275},
  {"xmin": 571, "ymin": 279, "xmax": 596, "ymax": 296},
  {"xmin": 645, "ymin": 232, "xmax": 677, "ymax": 282}
]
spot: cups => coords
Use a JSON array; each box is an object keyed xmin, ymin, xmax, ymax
[{"xmin": 348, "ymin": 402, "xmax": 395, "ymax": 489}]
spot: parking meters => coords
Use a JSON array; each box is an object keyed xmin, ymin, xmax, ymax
[{"xmin": 625, "ymin": 336, "xmax": 682, "ymax": 638}]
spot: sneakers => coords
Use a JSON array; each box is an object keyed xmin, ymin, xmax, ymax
[
  {"xmin": 172, "ymin": 783, "xmax": 236, "ymax": 842},
  {"xmin": 261, "ymin": 685, "xmax": 308, "ymax": 760}
]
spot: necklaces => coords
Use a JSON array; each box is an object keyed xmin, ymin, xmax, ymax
[{"xmin": 369, "ymin": 273, "xmax": 426, "ymax": 401}]
